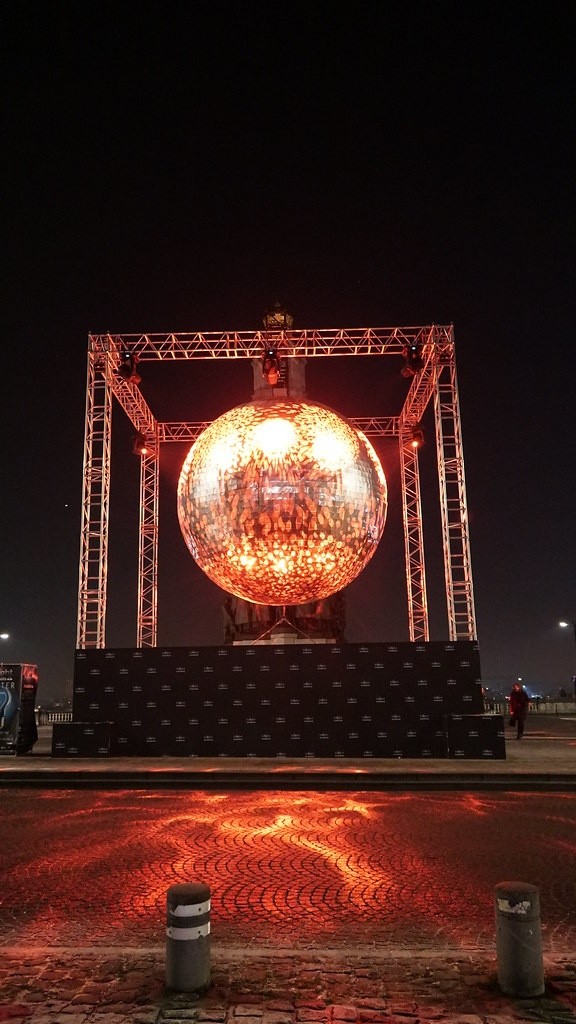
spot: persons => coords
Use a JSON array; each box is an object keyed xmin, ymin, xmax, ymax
[{"xmin": 510, "ymin": 683, "xmax": 528, "ymax": 740}]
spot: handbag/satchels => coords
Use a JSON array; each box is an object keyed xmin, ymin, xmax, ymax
[{"xmin": 508, "ymin": 714, "xmax": 516, "ymax": 727}]
